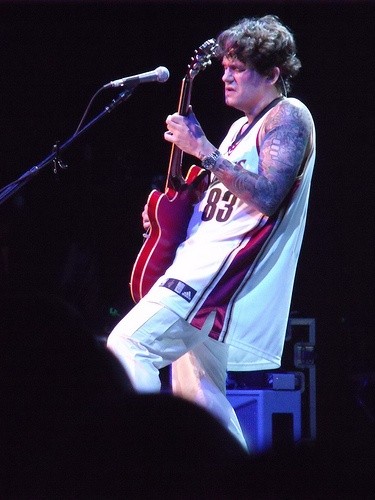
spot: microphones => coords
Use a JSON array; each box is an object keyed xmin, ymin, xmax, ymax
[{"xmin": 103, "ymin": 66, "xmax": 170, "ymax": 89}]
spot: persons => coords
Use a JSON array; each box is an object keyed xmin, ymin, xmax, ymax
[{"xmin": 103, "ymin": 14, "xmax": 317, "ymax": 451}]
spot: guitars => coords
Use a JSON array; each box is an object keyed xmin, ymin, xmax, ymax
[{"xmin": 130, "ymin": 37, "xmax": 224, "ymax": 304}]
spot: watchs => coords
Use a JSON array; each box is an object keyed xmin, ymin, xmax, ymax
[{"xmin": 201, "ymin": 150, "xmax": 221, "ymax": 172}]
